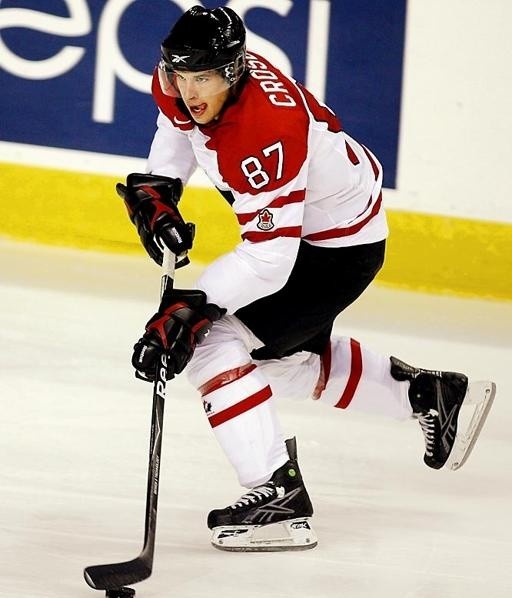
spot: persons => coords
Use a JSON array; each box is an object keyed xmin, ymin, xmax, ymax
[{"xmin": 113, "ymin": 3, "xmax": 470, "ymax": 530}]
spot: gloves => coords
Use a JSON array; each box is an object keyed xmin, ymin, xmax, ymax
[
  {"xmin": 116, "ymin": 173, "xmax": 196, "ymax": 269},
  {"xmin": 131, "ymin": 287, "xmax": 227, "ymax": 382}
]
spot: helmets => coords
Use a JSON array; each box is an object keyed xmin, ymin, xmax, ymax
[{"xmin": 157, "ymin": 5, "xmax": 247, "ymax": 99}]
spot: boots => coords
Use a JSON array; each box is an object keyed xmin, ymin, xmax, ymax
[
  {"xmin": 206, "ymin": 436, "xmax": 313, "ymax": 529},
  {"xmin": 389, "ymin": 355, "xmax": 467, "ymax": 469}
]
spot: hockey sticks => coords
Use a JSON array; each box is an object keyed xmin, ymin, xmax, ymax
[{"xmin": 84, "ymin": 231, "xmax": 175, "ymax": 588}]
